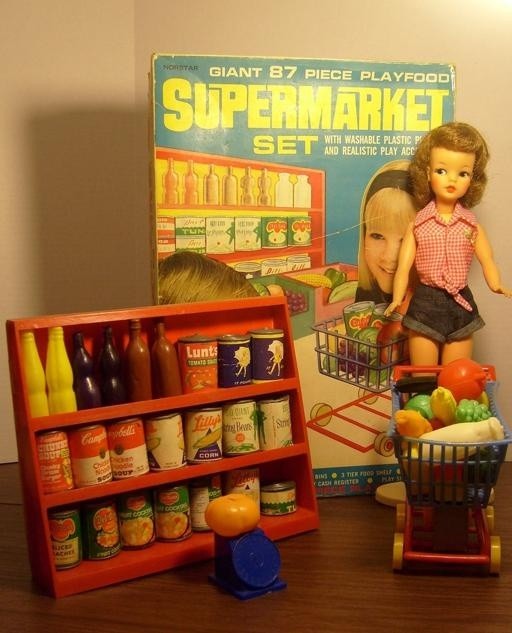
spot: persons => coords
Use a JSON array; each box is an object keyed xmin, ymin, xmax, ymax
[
  {"xmin": 159, "ymin": 251, "xmax": 260, "ymax": 304},
  {"xmin": 355, "ymin": 159, "xmax": 419, "ymax": 303},
  {"xmin": 383, "ymin": 122, "xmax": 512, "ymax": 377}
]
